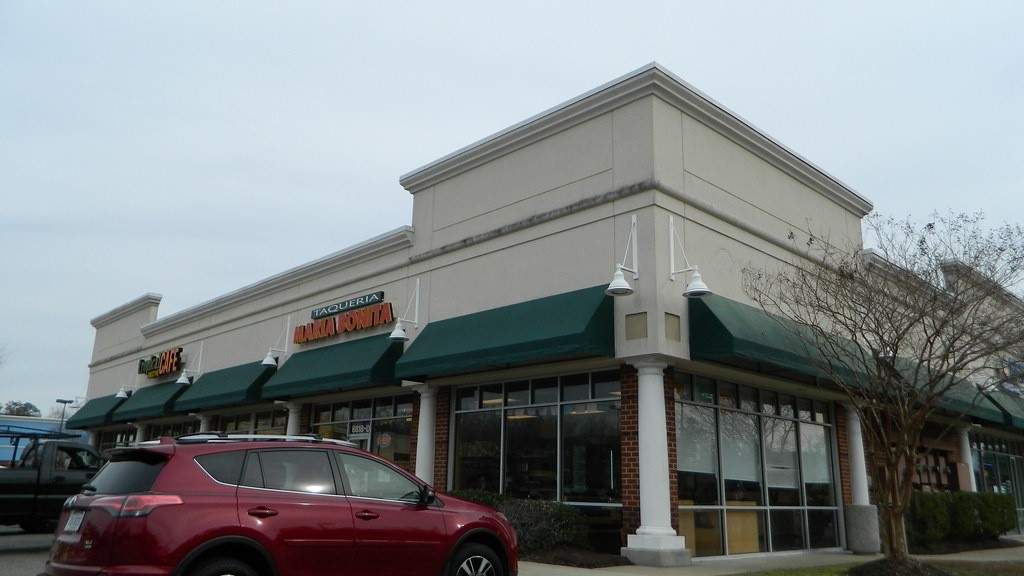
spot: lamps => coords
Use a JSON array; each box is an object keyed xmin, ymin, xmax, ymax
[
  {"xmin": 115, "ymin": 384, "xmax": 136, "ymax": 399},
  {"xmin": 602, "ymin": 214, "xmax": 640, "ymax": 297},
  {"xmin": 174, "ymin": 341, "xmax": 204, "ymax": 384},
  {"xmin": 261, "ymin": 315, "xmax": 291, "ymax": 367},
  {"xmin": 668, "ymin": 215, "xmax": 713, "ymax": 299},
  {"xmin": 385, "ymin": 277, "xmax": 421, "ymax": 342}
]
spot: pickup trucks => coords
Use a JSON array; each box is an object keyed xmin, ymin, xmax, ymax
[{"xmin": 0, "ymin": 439, "xmax": 107, "ymax": 535}]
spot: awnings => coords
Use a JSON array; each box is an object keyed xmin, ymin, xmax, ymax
[
  {"xmin": 876, "ymin": 356, "xmax": 1003, "ymax": 426},
  {"xmin": 112, "ymin": 376, "xmax": 193, "ymax": 421},
  {"xmin": 66, "ymin": 390, "xmax": 132, "ymax": 429},
  {"xmin": 394, "ymin": 284, "xmax": 616, "ymax": 384},
  {"xmin": 975, "ymin": 382, "xmax": 1024, "ymax": 430},
  {"xmin": 260, "ymin": 329, "xmax": 404, "ymax": 401},
  {"xmin": 173, "ymin": 357, "xmax": 279, "ymax": 413},
  {"xmin": 688, "ymin": 291, "xmax": 901, "ymax": 404}
]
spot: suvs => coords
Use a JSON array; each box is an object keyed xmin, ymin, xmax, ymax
[{"xmin": 44, "ymin": 430, "xmax": 518, "ymax": 576}]
